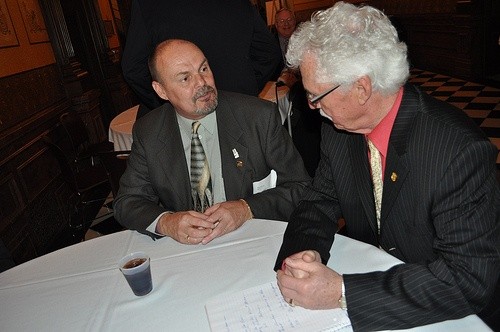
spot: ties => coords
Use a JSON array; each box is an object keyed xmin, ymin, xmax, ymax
[
  {"xmin": 366, "ymin": 139, "xmax": 384, "ymax": 235},
  {"xmin": 191, "ymin": 121, "xmax": 213, "ymax": 213},
  {"xmin": 284, "ymin": 39, "xmax": 291, "ymax": 69}
]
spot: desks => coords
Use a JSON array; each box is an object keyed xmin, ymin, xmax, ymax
[
  {"xmin": 107, "ymin": 80, "xmax": 292, "ymax": 164},
  {"xmin": 0, "ymin": 218, "xmax": 492, "ymax": 331}
]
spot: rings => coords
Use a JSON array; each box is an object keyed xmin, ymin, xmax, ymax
[
  {"xmin": 289, "ymin": 298, "xmax": 294, "ymax": 307},
  {"xmin": 186, "ymin": 235, "xmax": 190, "ymax": 240}
]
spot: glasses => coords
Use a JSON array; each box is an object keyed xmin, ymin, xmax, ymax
[{"xmin": 305, "ymin": 83, "xmax": 340, "ymax": 108}]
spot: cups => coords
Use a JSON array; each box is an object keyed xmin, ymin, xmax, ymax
[{"xmin": 118, "ymin": 251, "xmax": 153, "ymax": 297}]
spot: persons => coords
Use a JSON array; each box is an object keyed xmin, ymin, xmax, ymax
[
  {"xmin": 113, "ymin": 37, "xmax": 314, "ymax": 245},
  {"xmin": 117, "ymin": 0, "xmax": 322, "ymax": 120},
  {"xmin": 274, "ymin": 2, "xmax": 500, "ymax": 332}
]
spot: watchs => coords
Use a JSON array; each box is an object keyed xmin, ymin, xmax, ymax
[{"xmin": 338, "ymin": 281, "xmax": 346, "ymax": 310}]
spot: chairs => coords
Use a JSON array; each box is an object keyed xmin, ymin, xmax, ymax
[
  {"xmin": 46, "ymin": 123, "xmax": 115, "ymax": 228},
  {"xmin": 61, "ymin": 111, "xmax": 116, "ymax": 191}
]
